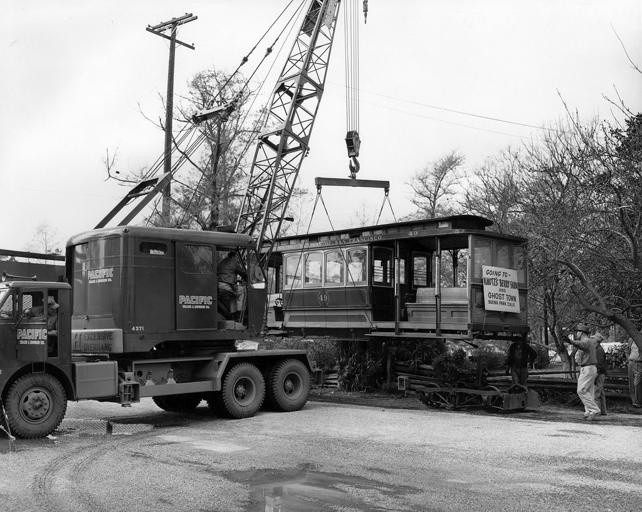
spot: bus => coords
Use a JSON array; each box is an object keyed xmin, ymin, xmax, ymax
[{"xmin": 254, "ymin": 209, "xmax": 533, "ymax": 346}]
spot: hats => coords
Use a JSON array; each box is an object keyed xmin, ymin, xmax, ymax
[{"xmin": 574, "ymin": 324, "xmax": 589, "ymax": 334}]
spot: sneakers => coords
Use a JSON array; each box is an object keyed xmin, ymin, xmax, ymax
[
  {"xmin": 629, "ymin": 405, "xmax": 642, "ymax": 412},
  {"xmin": 584, "ymin": 410, "xmax": 601, "ymax": 421}
]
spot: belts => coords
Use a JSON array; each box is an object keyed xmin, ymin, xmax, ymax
[
  {"xmin": 630, "ymin": 359, "xmax": 642, "ymax": 363},
  {"xmin": 580, "ymin": 364, "xmax": 594, "ymax": 367}
]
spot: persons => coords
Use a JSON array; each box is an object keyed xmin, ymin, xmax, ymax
[
  {"xmin": 625, "ymin": 326, "xmax": 642, "ymax": 409},
  {"xmin": 0, "ymin": 294, "xmax": 60, "ymax": 358},
  {"xmin": 561, "ymin": 322, "xmax": 602, "ymax": 421},
  {"xmin": 219, "ymin": 248, "xmax": 249, "ymax": 324},
  {"xmin": 590, "ymin": 331, "xmax": 609, "ymax": 415},
  {"xmin": 504, "ymin": 338, "xmax": 537, "ymax": 389}
]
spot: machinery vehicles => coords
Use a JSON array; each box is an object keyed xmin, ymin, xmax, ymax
[{"xmin": 2, "ymin": 0, "xmax": 371, "ymax": 453}]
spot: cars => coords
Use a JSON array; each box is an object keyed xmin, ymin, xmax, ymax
[{"xmin": 444, "ymin": 338, "xmax": 628, "ymax": 365}]
